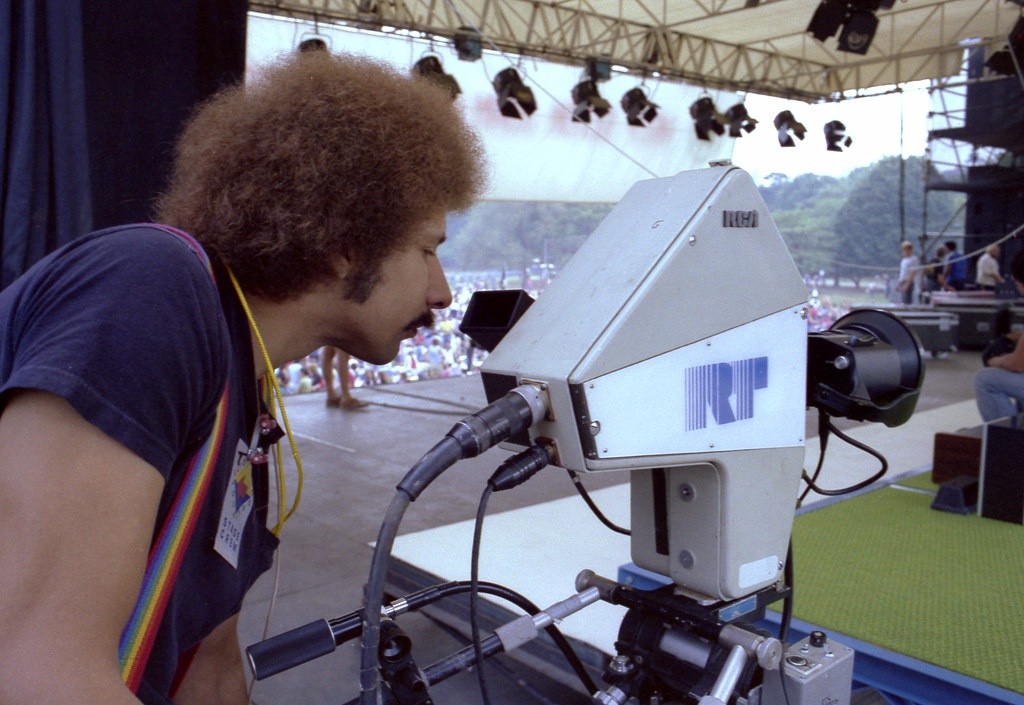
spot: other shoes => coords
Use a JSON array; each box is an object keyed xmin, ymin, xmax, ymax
[
  {"xmin": 325, "ymin": 394, "xmax": 338, "ymax": 406},
  {"xmin": 337, "ymin": 397, "xmax": 368, "ymax": 410}
]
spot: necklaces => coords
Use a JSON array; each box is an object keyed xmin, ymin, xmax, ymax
[{"xmin": 222, "ymin": 262, "xmax": 303, "ymax": 536}]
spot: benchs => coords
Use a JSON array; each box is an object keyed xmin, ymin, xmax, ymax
[{"xmin": 933, "ymin": 410, "xmax": 1024, "ymax": 526}]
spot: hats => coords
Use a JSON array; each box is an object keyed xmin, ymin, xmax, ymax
[{"xmin": 900, "ymin": 240, "xmax": 912, "ymax": 249}]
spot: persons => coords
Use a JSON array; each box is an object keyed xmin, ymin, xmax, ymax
[
  {"xmin": 974, "ymin": 241, "xmax": 1024, "ymax": 421},
  {"xmin": 273, "ymin": 258, "xmax": 554, "ymax": 397},
  {"xmin": 322, "ymin": 344, "xmax": 371, "ymax": 409},
  {"xmin": 896, "ymin": 240, "xmax": 1005, "ymax": 304},
  {"xmin": 0, "ymin": 47, "xmax": 484, "ymax": 705},
  {"xmin": 808, "ymin": 287, "xmax": 843, "ymax": 332}
]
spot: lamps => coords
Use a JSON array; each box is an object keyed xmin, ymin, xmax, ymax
[{"xmin": 296, "ymin": 17, "xmax": 854, "ymax": 154}]
